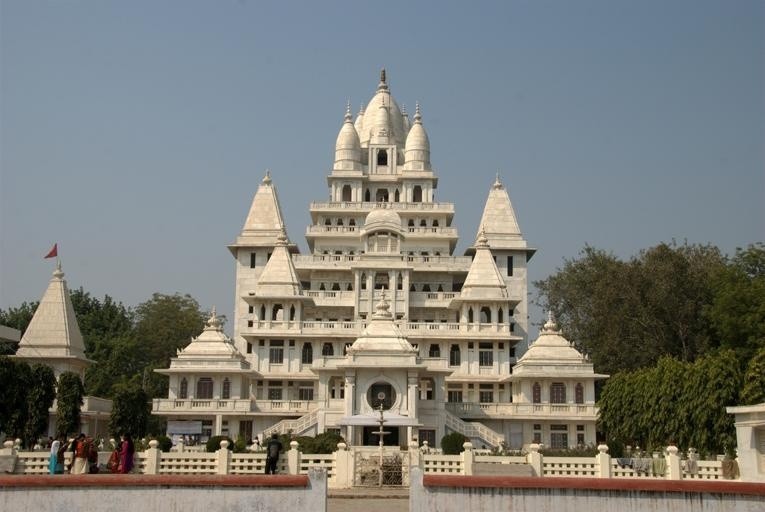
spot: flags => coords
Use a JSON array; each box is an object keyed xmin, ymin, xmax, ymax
[{"xmin": 45, "ymin": 243, "xmax": 57, "ymax": 258}]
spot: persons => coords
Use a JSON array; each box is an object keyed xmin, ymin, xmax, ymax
[
  {"xmin": 265, "ymin": 434, "xmax": 282, "ymax": 475},
  {"xmin": 26, "ymin": 433, "xmax": 130, "ymax": 475}
]
